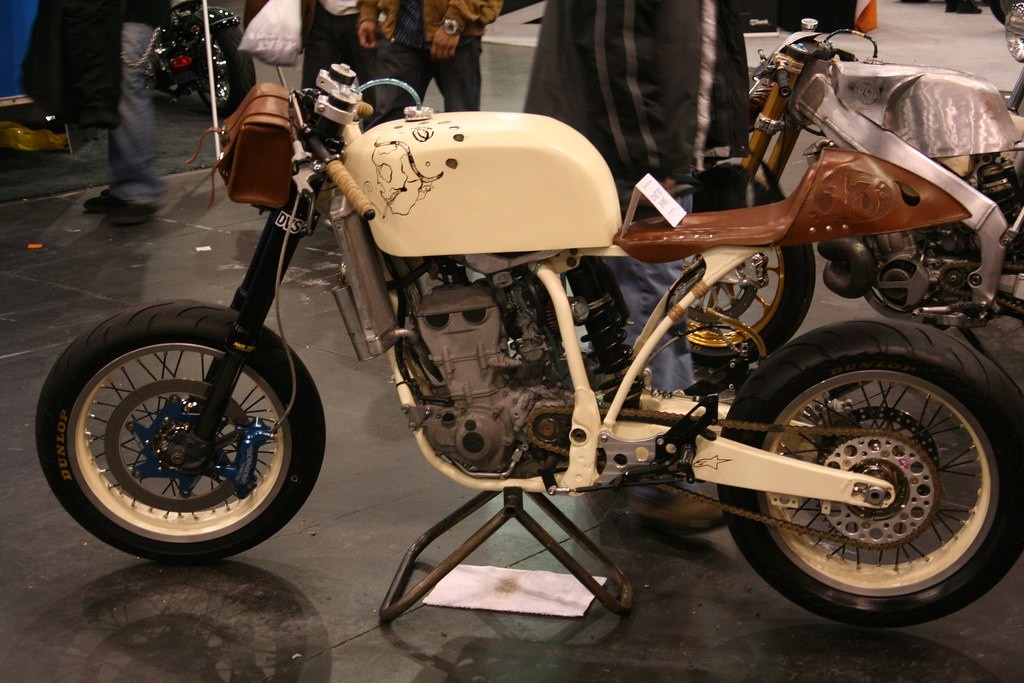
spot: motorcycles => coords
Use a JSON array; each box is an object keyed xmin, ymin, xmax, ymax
[
  {"xmin": 31, "ymin": 62, "xmax": 1023, "ymax": 628},
  {"xmin": 681, "ymin": 17, "xmax": 1023, "ymax": 373},
  {"xmin": 149, "ymin": 0, "xmax": 258, "ymax": 119}
]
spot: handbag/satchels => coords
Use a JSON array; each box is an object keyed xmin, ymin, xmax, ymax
[
  {"xmin": 237, "ymin": 0, "xmax": 301, "ymax": 66},
  {"xmin": 185, "ymin": 83, "xmax": 294, "ymax": 210}
]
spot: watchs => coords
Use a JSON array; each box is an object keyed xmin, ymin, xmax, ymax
[{"xmin": 443, "ymin": 19, "xmax": 461, "ymax": 36}]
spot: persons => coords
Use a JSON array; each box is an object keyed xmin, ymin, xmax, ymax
[
  {"xmin": 524, "ymin": 0, "xmax": 752, "ymax": 535},
  {"xmin": 298, "ymin": 0, "xmax": 503, "ymax": 229},
  {"xmin": 83, "ymin": 0, "xmax": 169, "ymax": 225}
]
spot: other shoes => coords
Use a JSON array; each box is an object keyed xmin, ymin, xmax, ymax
[
  {"xmin": 109, "ymin": 201, "xmax": 166, "ymax": 222},
  {"xmin": 83, "ymin": 189, "xmax": 127, "ymax": 212},
  {"xmin": 626, "ymin": 482, "xmax": 725, "ymax": 533},
  {"xmin": 945, "ymin": 2, "xmax": 983, "ymax": 15}
]
